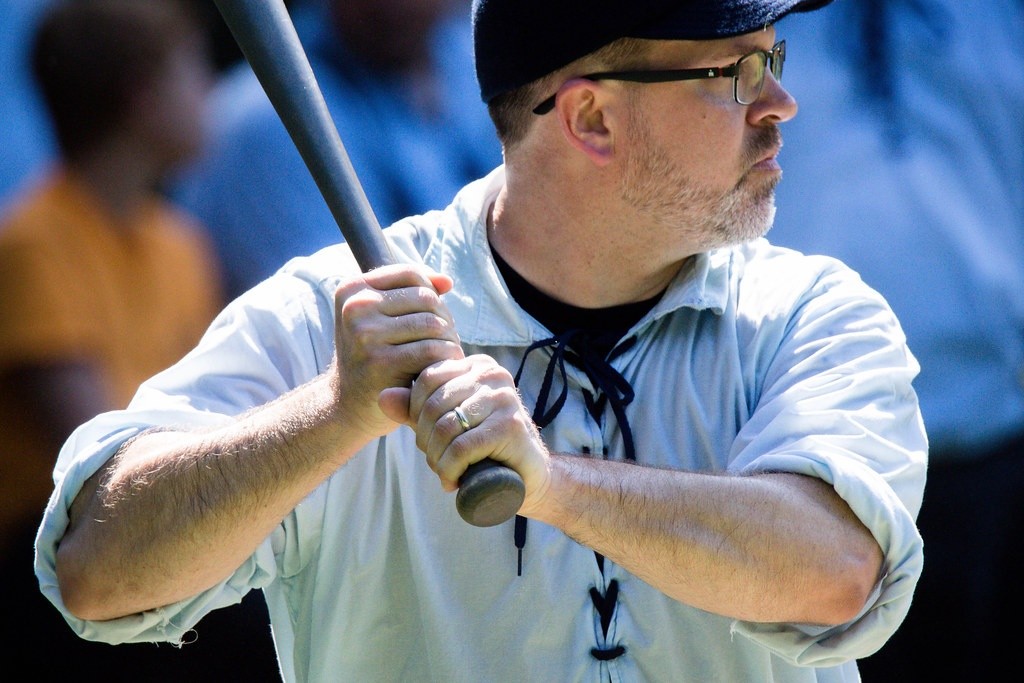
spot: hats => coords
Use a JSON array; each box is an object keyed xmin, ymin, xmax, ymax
[{"xmin": 471, "ymin": 0, "xmax": 834, "ymax": 102}]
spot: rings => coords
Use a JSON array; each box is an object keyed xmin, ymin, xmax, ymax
[{"xmin": 454, "ymin": 406, "xmax": 471, "ymax": 430}]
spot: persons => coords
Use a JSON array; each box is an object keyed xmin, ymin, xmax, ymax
[
  {"xmin": 1, "ymin": 0, "xmax": 1023, "ymax": 682},
  {"xmin": 30, "ymin": 0, "xmax": 929, "ymax": 683}
]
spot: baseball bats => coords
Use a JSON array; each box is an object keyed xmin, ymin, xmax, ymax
[{"xmin": 221, "ymin": 0, "xmax": 530, "ymax": 530}]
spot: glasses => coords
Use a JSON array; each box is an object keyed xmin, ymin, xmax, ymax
[{"xmin": 532, "ymin": 40, "xmax": 786, "ymax": 116}]
